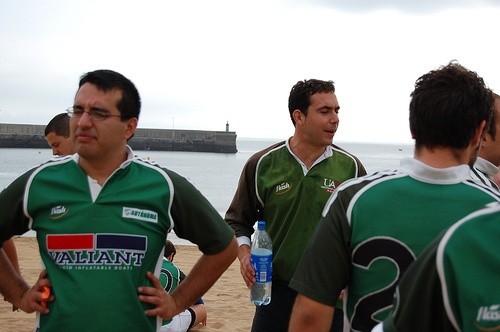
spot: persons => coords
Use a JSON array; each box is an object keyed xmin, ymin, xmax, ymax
[
  {"xmin": 0, "ymin": 70, "xmax": 239, "ymax": 332},
  {"xmin": 288, "ymin": 58, "xmax": 500, "ymax": 332},
  {"xmin": 224, "ymin": 79, "xmax": 368, "ymax": 332}
]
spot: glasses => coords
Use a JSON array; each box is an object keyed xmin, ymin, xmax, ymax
[{"xmin": 65, "ymin": 106, "xmax": 121, "ymax": 122}]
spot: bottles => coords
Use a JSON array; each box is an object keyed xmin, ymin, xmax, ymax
[{"xmin": 249, "ymin": 222, "xmax": 274, "ymax": 305}]
